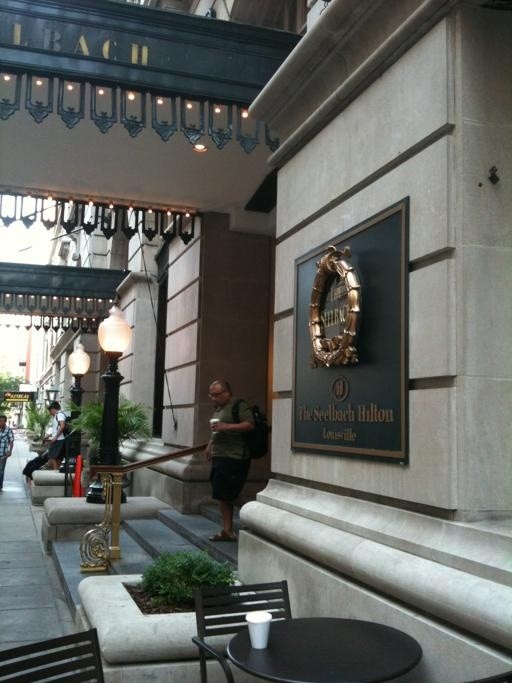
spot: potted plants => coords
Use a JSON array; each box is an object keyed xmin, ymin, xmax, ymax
[{"xmin": 73, "ymin": 546, "xmax": 283, "ymax": 682}]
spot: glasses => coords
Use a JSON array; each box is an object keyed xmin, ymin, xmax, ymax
[{"xmin": 208, "ymin": 391, "xmax": 223, "ymax": 397}]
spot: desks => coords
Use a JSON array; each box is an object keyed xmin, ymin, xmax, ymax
[{"xmin": 225, "ymin": 616, "xmax": 422, "ymax": 683}]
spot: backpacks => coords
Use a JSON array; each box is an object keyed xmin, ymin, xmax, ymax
[
  {"xmin": 232, "ymin": 399, "xmax": 271, "ymax": 458},
  {"xmin": 56, "ymin": 412, "xmax": 72, "ymax": 437}
]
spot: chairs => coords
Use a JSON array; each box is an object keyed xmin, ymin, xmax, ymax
[{"xmin": 0, "ymin": 580, "xmax": 291, "ymax": 683}]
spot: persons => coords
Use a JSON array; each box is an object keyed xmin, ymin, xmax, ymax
[
  {"xmin": 47, "ymin": 405, "xmax": 67, "ymax": 470},
  {"xmin": 0, "ymin": 414, "xmax": 14, "ymax": 491},
  {"xmin": 203, "ymin": 377, "xmax": 257, "ymax": 541},
  {"xmin": 50, "ymin": 401, "xmax": 69, "ymax": 421}
]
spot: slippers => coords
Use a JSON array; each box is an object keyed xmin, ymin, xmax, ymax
[{"xmin": 209, "ymin": 530, "xmax": 236, "ymax": 542}]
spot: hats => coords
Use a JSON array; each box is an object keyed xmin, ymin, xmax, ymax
[{"xmin": 47, "ymin": 401, "xmax": 59, "ymax": 409}]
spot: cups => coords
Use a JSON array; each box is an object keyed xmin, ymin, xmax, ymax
[
  {"xmin": 209, "ymin": 417, "xmax": 221, "ymax": 433},
  {"xmin": 244, "ymin": 610, "xmax": 273, "ymax": 649}
]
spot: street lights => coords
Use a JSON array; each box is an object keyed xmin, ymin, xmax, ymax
[
  {"xmin": 87, "ymin": 305, "xmax": 131, "ymax": 503},
  {"xmin": 59, "ymin": 344, "xmax": 90, "ymax": 474}
]
